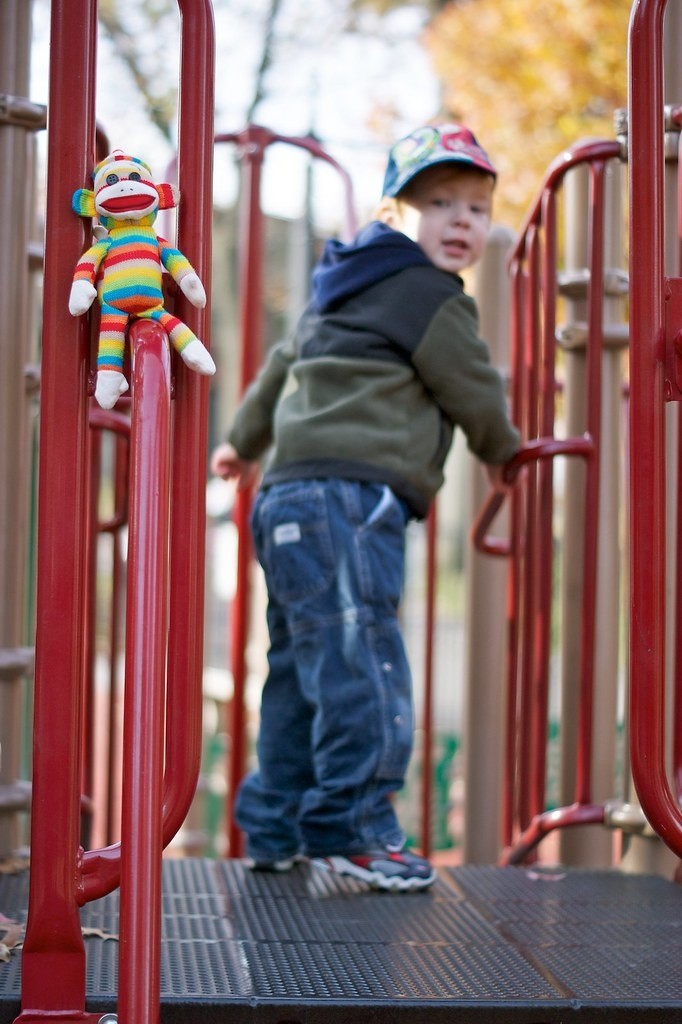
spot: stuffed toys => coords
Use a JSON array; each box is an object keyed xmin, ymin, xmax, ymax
[{"xmin": 69, "ymin": 150, "xmax": 215, "ymax": 409}]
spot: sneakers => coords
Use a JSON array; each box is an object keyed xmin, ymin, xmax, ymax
[
  {"xmin": 243, "ymin": 852, "xmax": 302, "ymax": 872},
  {"xmin": 311, "ymin": 847, "xmax": 438, "ymax": 893}
]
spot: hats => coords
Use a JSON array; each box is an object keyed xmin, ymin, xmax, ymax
[{"xmin": 381, "ymin": 122, "xmax": 497, "ymax": 199}]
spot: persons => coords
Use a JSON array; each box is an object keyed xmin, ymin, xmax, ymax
[{"xmin": 212, "ymin": 121, "xmax": 520, "ymax": 891}]
